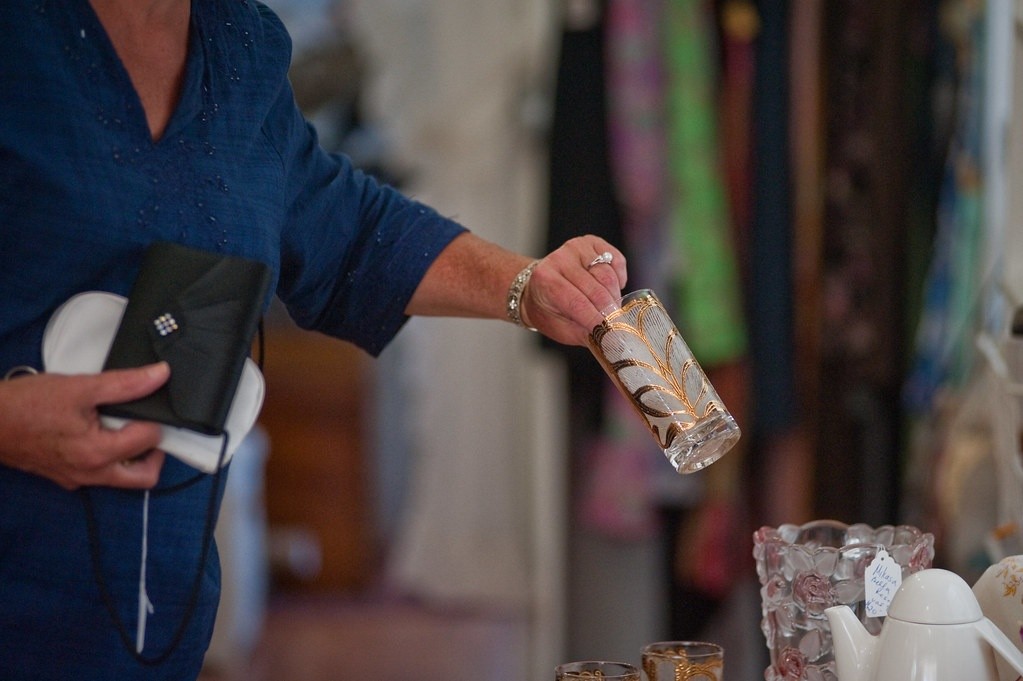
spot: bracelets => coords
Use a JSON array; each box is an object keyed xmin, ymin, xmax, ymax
[{"xmin": 506, "ymin": 259, "xmax": 541, "ymax": 332}]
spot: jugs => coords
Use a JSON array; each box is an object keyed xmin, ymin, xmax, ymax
[{"xmin": 825, "ymin": 567, "xmax": 1022, "ymax": 681}]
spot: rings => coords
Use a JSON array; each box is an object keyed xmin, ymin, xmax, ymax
[{"xmin": 587, "ymin": 252, "xmax": 612, "ymax": 271}]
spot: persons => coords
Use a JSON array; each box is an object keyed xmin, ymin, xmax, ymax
[{"xmin": 0, "ymin": 0, "xmax": 628, "ymax": 681}]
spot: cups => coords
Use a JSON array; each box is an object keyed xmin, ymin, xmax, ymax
[
  {"xmin": 552, "ymin": 661, "xmax": 643, "ymax": 680},
  {"xmin": 753, "ymin": 520, "xmax": 936, "ymax": 680},
  {"xmin": 578, "ymin": 289, "xmax": 740, "ymax": 473},
  {"xmin": 639, "ymin": 642, "xmax": 724, "ymax": 680}
]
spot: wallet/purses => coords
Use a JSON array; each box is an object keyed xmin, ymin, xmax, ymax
[{"xmin": 94, "ymin": 241, "xmax": 271, "ymax": 436}]
